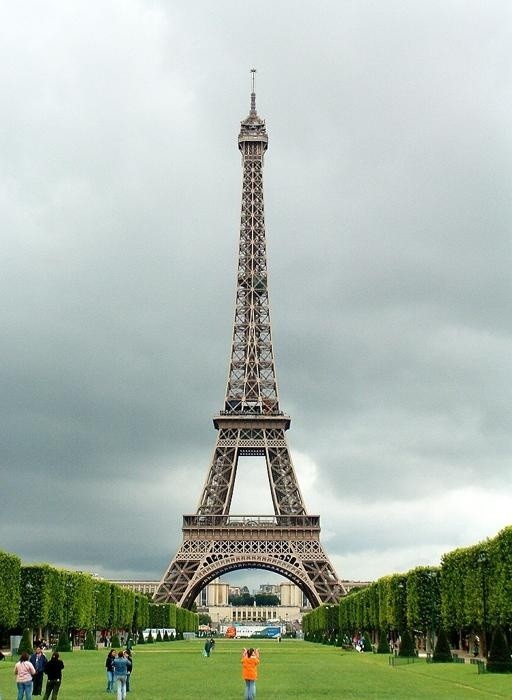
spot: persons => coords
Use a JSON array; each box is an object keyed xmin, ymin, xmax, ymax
[
  {"xmin": 14, "ymin": 652, "xmax": 37, "ymax": 700},
  {"xmin": 44, "ymin": 652, "xmax": 65, "ymax": 700},
  {"xmin": 30, "ymin": 647, "xmax": 49, "ymax": 695},
  {"xmin": 241, "ymin": 649, "xmax": 261, "ymax": 700},
  {"xmin": 106, "ymin": 649, "xmax": 116, "ymax": 690},
  {"xmin": 128, "ymin": 637, "xmax": 133, "ymax": 652},
  {"xmin": 210, "ymin": 639, "xmax": 215, "ymax": 650},
  {"xmin": 112, "ymin": 651, "xmax": 132, "ymax": 700},
  {"xmin": 205, "ymin": 640, "xmax": 212, "ymax": 656},
  {"xmin": 243, "ymin": 647, "xmax": 257, "ymax": 657},
  {"xmin": 124, "ymin": 650, "xmax": 133, "ymax": 691}
]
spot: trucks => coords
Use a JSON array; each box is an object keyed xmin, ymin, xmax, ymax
[{"xmin": 224, "ymin": 625, "xmax": 281, "ymax": 639}]
